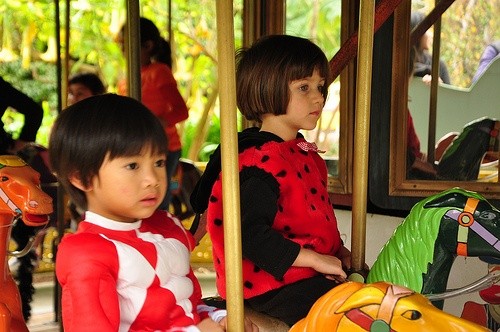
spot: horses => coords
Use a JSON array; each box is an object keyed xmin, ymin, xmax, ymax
[
  {"xmin": 0, "ymin": 155, "xmax": 55, "ymax": 332},
  {"xmin": 348, "ymin": 187, "xmax": 500, "ymax": 294},
  {"xmin": 171, "ymin": 162, "xmax": 202, "ymax": 220},
  {"xmin": 413, "ymin": 117, "xmax": 500, "ymax": 181},
  {"xmin": 288, "ymin": 281, "xmax": 497, "ymax": 332}
]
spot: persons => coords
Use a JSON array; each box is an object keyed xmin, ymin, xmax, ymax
[
  {"xmin": 411, "ymin": 13, "xmax": 451, "ymax": 85},
  {"xmin": 49, "ymin": 94, "xmax": 259, "ymax": 332},
  {"xmin": 0, "ymin": 17, "xmax": 189, "ymax": 161},
  {"xmin": 207, "ymin": 35, "xmax": 369, "ymax": 328}
]
